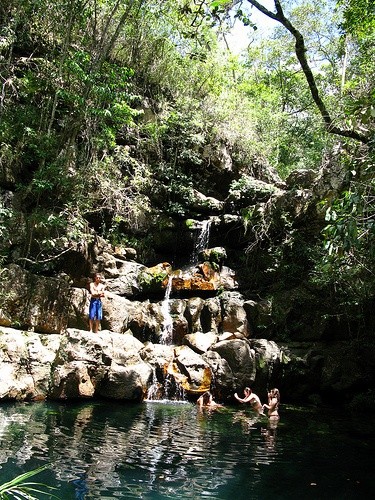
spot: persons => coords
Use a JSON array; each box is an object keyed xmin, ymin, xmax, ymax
[
  {"xmin": 234, "ymin": 387, "xmax": 263, "ymax": 417},
  {"xmin": 262, "ymin": 388, "xmax": 281, "ymax": 449},
  {"xmin": 88, "ymin": 272, "xmax": 105, "ymax": 333},
  {"xmin": 203, "ymin": 393, "xmax": 224, "ymax": 414},
  {"xmin": 195, "ymin": 391, "xmax": 209, "ymax": 415}
]
